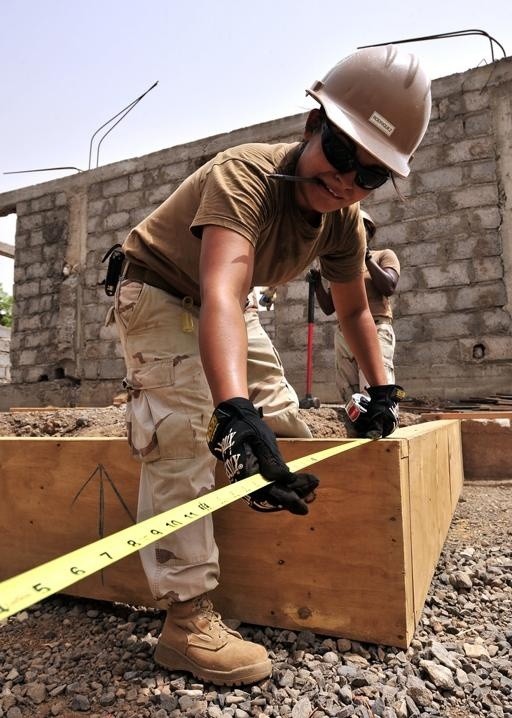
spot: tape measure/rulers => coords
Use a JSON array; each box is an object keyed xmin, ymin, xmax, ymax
[{"xmin": 0, "ymin": 392, "xmax": 384, "ymax": 624}]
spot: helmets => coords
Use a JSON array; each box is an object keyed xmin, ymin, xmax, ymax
[
  {"xmin": 360, "ymin": 209, "xmax": 376, "ymax": 234},
  {"xmin": 306, "ymin": 44, "xmax": 432, "ymax": 180}
]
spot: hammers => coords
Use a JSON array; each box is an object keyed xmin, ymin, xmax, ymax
[{"xmin": 298, "ymin": 272, "xmax": 320, "ymax": 409}]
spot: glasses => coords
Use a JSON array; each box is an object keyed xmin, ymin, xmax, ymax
[{"xmin": 321, "ymin": 110, "xmax": 387, "ymax": 190}]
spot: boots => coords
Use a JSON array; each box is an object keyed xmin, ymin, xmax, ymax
[{"xmin": 154, "ymin": 592, "xmax": 272, "ymax": 687}]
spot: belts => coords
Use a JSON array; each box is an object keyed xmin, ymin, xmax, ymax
[{"xmin": 120, "ymin": 261, "xmax": 184, "ymax": 299}]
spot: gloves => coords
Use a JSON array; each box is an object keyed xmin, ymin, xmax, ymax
[
  {"xmin": 349, "ymin": 385, "xmax": 405, "ymax": 438},
  {"xmin": 206, "ymin": 398, "xmax": 319, "ymax": 515}
]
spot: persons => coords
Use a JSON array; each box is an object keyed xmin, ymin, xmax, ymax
[
  {"xmin": 305, "ymin": 209, "xmax": 401, "ymax": 440},
  {"xmin": 106, "ymin": 44, "xmax": 433, "ymax": 688}
]
